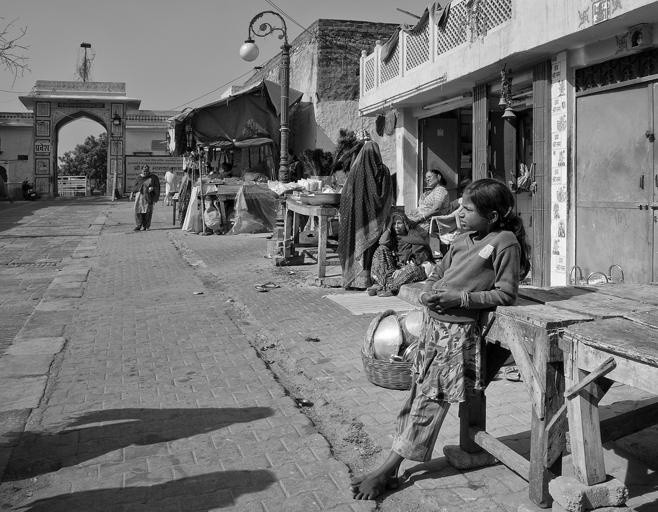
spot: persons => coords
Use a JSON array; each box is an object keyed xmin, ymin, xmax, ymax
[
  {"xmin": 350, "ymin": 176, "xmax": 533, "ymax": 501},
  {"xmin": 130, "ymin": 162, "xmax": 242, "ymax": 231},
  {"xmin": 287, "ymin": 149, "xmax": 304, "ymax": 182},
  {"xmin": 339, "ymin": 139, "xmax": 467, "ymax": 295}
]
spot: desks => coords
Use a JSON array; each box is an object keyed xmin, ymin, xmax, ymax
[
  {"xmin": 458, "ymin": 279, "xmax": 657, "ymax": 509},
  {"xmin": 560, "ymin": 309, "xmax": 657, "ymax": 488},
  {"xmin": 163, "ymin": 168, "xmax": 283, "ymax": 233},
  {"xmin": 282, "ymin": 196, "xmax": 406, "ymax": 277}
]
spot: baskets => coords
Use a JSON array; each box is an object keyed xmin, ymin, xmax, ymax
[{"xmin": 361, "ymin": 309, "xmax": 413, "ymax": 390}]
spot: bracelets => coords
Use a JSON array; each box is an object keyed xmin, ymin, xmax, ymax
[{"xmin": 460, "ymin": 292, "xmax": 469, "ymax": 308}]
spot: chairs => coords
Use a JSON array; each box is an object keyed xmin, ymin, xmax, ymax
[{"xmin": 420, "ymin": 195, "xmax": 465, "ymax": 244}]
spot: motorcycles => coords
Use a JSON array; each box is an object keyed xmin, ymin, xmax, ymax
[{"xmin": 20, "ymin": 176, "xmax": 37, "ymax": 201}]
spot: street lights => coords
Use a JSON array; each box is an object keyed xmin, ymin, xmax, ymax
[{"xmin": 239, "ymin": 10, "xmax": 298, "ymax": 260}]
